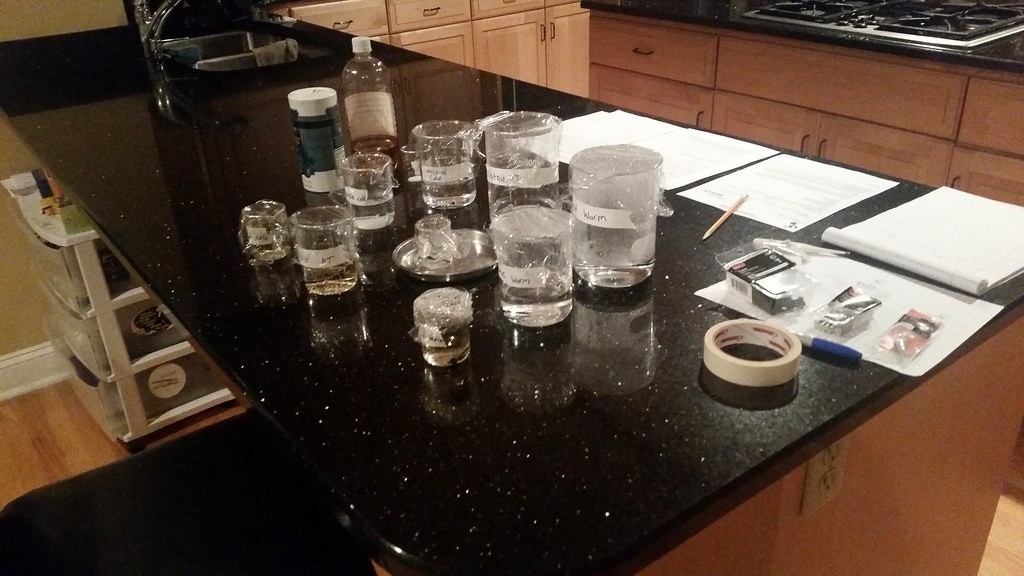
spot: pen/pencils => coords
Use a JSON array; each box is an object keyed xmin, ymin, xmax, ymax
[
  {"xmin": 703, "ymin": 191, "xmax": 751, "ymax": 242},
  {"xmin": 792, "ymin": 333, "xmax": 862, "ymax": 365}
]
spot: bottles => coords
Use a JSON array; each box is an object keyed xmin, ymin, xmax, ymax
[
  {"xmin": 343, "ymin": 37, "xmax": 399, "ymax": 170},
  {"xmin": 287, "ymin": 87, "xmax": 347, "ymax": 192}
]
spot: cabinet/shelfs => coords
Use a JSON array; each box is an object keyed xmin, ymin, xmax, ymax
[
  {"xmin": 1, "ymin": 167, "xmax": 235, "ymax": 445},
  {"xmin": 280, "ymin": 0, "xmax": 1023, "ymax": 208}
]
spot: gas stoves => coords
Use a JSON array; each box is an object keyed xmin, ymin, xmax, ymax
[{"xmin": 740, "ymin": 0, "xmax": 1024, "ymax": 47}]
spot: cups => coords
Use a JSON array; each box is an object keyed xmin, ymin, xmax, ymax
[
  {"xmin": 483, "ymin": 111, "xmax": 561, "ymax": 221},
  {"xmin": 413, "ymin": 287, "xmax": 472, "ymax": 367},
  {"xmin": 290, "ymin": 205, "xmax": 359, "ymax": 295},
  {"xmin": 340, "ymin": 153, "xmax": 395, "ymax": 230},
  {"xmin": 412, "ymin": 121, "xmax": 476, "ymax": 208},
  {"xmin": 488, "ymin": 205, "xmax": 576, "ymax": 326},
  {"xmin": 569, "ymin": 145, "xmax": 663, "ymax": 288},
  {"xmin": 401, "ymin": 145, "xmax": 435, "ymax": 182},
  {"xmin": 242, "ymin": 202, "xmax": 290, "ymax": 259},
  {"xmin": 414, "ymin": 215, "xmax": 451, "ymax": 270}
]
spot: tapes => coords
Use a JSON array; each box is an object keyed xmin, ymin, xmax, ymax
[{"xmin": 701, "ymin": 319, "xmax": 799, "ymax": 386}]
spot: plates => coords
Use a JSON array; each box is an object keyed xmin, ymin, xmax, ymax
[{"xmin": 392, "ymin": 228, "xmax": 499, "ymax": 282}]
